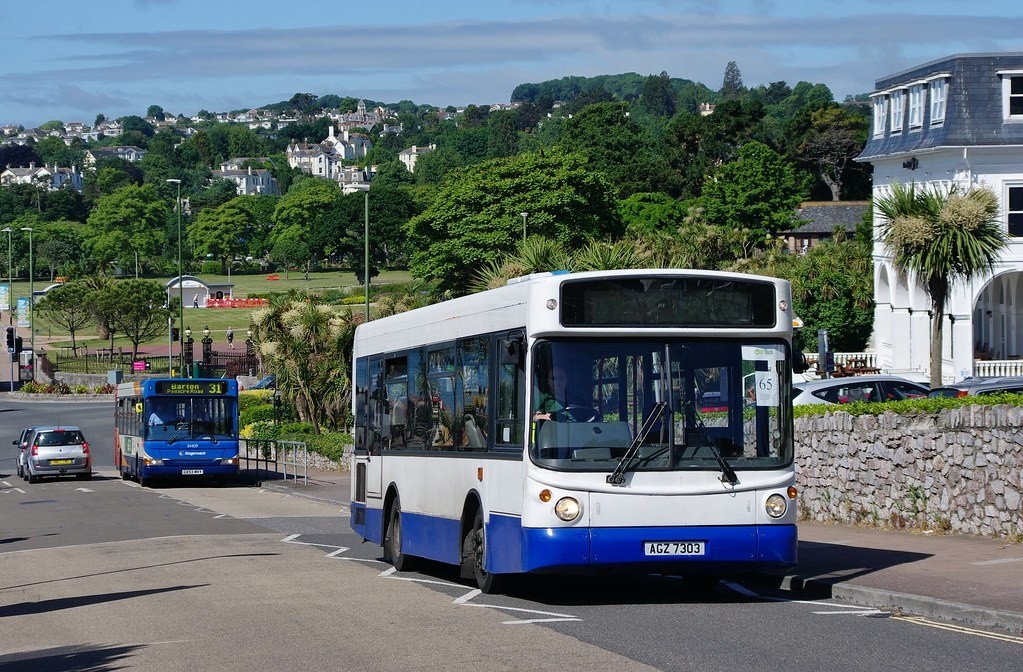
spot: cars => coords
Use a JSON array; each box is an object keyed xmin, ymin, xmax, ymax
[
  {"xmin": 12, "ymin": 424, "xmax": 92, "ymax": 484},
  {"xmin": 791, "ymin": 374, "xmax": 1023, "ymax": 408}
]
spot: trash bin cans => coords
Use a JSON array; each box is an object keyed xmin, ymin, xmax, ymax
[{"xmin": 193, "ymin": 360, "xmax": 204, "ymax": 378}]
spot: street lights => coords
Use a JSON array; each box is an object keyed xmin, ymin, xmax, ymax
[
  {"xmin": 343, "ymin": 183, "xmax": 372, "ymax": 324},
  {"xmin": 20, "ymin": 226, "xmax": 34, "ymax": 387},
  {"xmin": 520, "ymin": 212, "xmax": 529, "ymax": 275},
  {"xmin": 1, "ymin": 228, "xmax": 15, "ymax": 393},
  {"xmin": 165, "ymin": 178, "xmax": 185, "ymax": 379}
]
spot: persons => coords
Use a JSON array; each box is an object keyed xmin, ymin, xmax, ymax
[
  {"xmin": 225, "ymin": 326, "xmax": 235, "ymax": 350},
  {"xmin": 533, "ymin": 367, "xmax": 576, "ymax": 423},
  {"xmin": 149, "ymin": 404, "xmax": 172, "ymax": 426},
  {"xmin": 193, "ymin": 294, "xmax": 199, "ymax": 308}
]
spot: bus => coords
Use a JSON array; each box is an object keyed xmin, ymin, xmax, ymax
[
  {"xmin": 113, "ymin": 377, "xmax": 241, "ymax": 487},
  {"xmin": 348, "ymin": 267, "xmax": 811, "ymax": 596}
]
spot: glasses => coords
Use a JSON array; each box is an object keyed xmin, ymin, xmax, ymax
[{"xmin": 548, "ymin": 376, "xmax": 566, "ymax": 381}]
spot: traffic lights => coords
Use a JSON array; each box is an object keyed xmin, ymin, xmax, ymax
[
  {"xmin": 171, "ymin": 328, "xmax": 179, "ymax": 341},
  {"xmin": 5, "ymin": 326, "xmax": 16, "ymax": 353}
]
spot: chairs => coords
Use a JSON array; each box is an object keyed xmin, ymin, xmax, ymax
[{"xmin": 464, "ymin": 414, "xmax": 487, "ymax": 448}]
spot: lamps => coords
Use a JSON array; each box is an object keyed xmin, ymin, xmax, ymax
[
  {"xmin": 947, "ymin": 169, "xmax": 954, "ymax": 175},
  {"xmin": 869, "ymin": 178, "xmax": 878, "ymax": 181},
  {"xmin": 886, "ymin": 175, "xmax": 893, "ymax": 180},
  {"xmin": 925, "ymin": 171, "xmax": 932, "ymax": 176}
]
